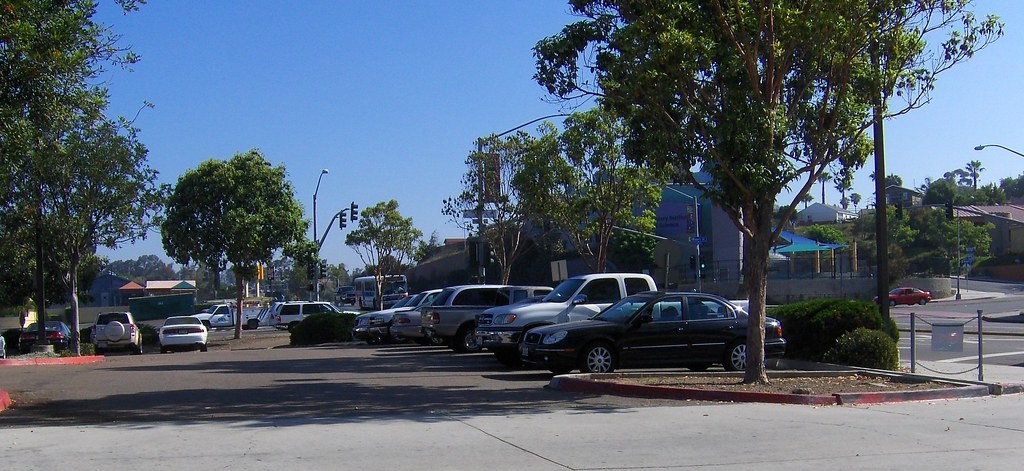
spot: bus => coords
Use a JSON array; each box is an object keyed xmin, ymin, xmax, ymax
[{"xmin": 354, "ymin": 274, "xmax": 409, "ymax": 311}]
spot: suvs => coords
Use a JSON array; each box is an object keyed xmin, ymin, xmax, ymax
[
  {"xmin": 268, "ymin": 299, "xmax": 362, "ymax": 333},
  {"xmin": 333, "ymin": 286, "xmax": 356, "ymax": 307},
  {"xmin": 90, "ymin": 312, "xmax": 143, "ymax": 355},
  {"xmin": 419, "ymin": 284, "xmax": 554, "ymax": 354}
]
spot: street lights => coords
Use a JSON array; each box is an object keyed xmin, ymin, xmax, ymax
[{"xmin": 313, "ymin": 169, "xmax": 329, "ymax": 299}]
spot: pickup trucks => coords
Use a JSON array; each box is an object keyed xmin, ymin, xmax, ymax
[
  {"xmin": 476, "ymin": 272, "xmax": 750, "ymax": 369},
  {"xmin": 191, "ymin": 303, "xmax": 272, "ymax": 330}
]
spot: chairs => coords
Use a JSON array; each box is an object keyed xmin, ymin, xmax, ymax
[{"xmin": 661, "ymin": 306, "xmax": 679, "ymax": 318}]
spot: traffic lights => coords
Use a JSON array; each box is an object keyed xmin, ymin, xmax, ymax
[
  {"xmin": 689, "ymin": 254, "xmax": 696, "ymax": 272},
  {"xmin": 700, "ymin": 255, "xmax": 707, "ymax": 271},
  {"xmin": 486, "ymin": 242, "xmax": 495, "ymax": 266},
  {"xmin": 351, "ymin": 203, "xmax": 358, "ymax": 221},
  {"xmin": 895, "ymin": 199, "xmax": 903, "ymax": 220},
  {"xmin": 944, "ymin": 203, "xmax": 954, "ymax": 221},
  {"xmin": 308, "ymin": 261, "xmax": 314, "ymax": 279},
  {"xmin": 339, "ymin": 212, "xmax": 346, "ymax": 229},
  {"xmin": 320, "ymin": 262, "xmax": 326, "ymax": 278}
]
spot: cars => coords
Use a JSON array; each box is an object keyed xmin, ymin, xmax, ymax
[
  {"xmin": 517, "ymin": 290, "xmax": 787, "ymax": 373},
  {"xmin": 2, "ymin": 320, "xmax": 72, "ymax": 355},
  {"xmin": 872, "ymin": 287, "xmax": 932, "ymax": 306},
  {"xmin": 351, "ymin": 288, "xmax": 447, "ymax": 346},
  {"xmin": 157, "ymin": 315, "xmax": 210, "ymax": 352}
]
